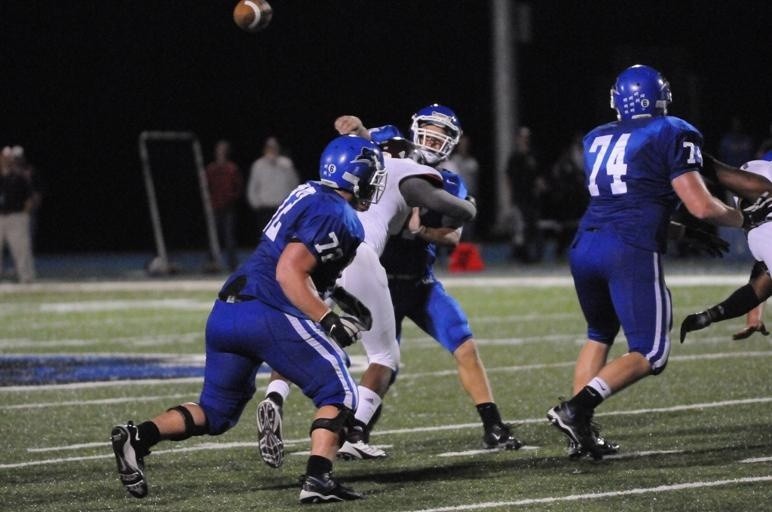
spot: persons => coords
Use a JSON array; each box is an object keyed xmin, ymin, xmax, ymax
[
  {"xmin": 547, "ymin": 63, "xmax": 772, "ymax": 460},
  {"xmin": 12, "ymin": 146, "xmax": 43, "ymax": 212},
  {"xmin": 334, "ymin": 103, "xmax": 525, "ymax": 450},
  {"xmin": 0, "ymin": 146, "xmax": 38, "ymax": 284},
  {"xmin": 111, "ymin": 134, "xmax": 387, "ymax": 504},
  {"xmin": 245, "ymin": 136, "xmax": 299, "ymax": 233},
  {"xmin": 205, "ymin": 139, "xmax": 245, "ymax": 272},
  {"xmin": 680, "ymin": 150, "xmax": 772, "ymax": 344},
  {"xmin": 733, "ymin": 261, "xmax": 769, "ymax": 340},
  {"xmin": 255, "ymin": 136, "xmax": 478, "ymax": 468}
]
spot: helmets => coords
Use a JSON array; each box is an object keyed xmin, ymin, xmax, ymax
[
  {"xmin": 610, "ymin": 65, "xmax": 672, "ymax": 122},
  {"xmin": 321, "ymin": 134, "xmax": 387, "ymax": 212},
  {"xmin": 409, "ymin": 104, "xmax": 464, "ymax": 167}
]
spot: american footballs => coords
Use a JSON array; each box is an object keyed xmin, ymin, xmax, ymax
[{"xmin": 233, "ymin": 0, "xmax": 273, "ymax": 30}]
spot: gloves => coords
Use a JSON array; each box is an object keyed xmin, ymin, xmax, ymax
[
  {"xmin": 742, "ymin": 191, "xmax": 772, "ymax": 230},
  {"xmin": 681, "ymin": 311, "xmax": 712, "ymax": 343},
  {"xmin": 318, "ymin": 308, "xmax": 363, "ymax": 347},
  {"xmin": 330, "ymin": 285, "xmax": 373, "ymax": 330}
]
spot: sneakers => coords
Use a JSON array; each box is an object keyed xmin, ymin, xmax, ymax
[
  {"xmin": 334, "ymin": 430, "xmax": 387, "ymax": 460},
  {"xmin": 299, "ymin": 472, "xmax": 361, "ymax": 504},
  {"xmin": 256, "ymin": 398, "xmax": 285, "ymax": 468},
  {"xmin": 480, "ymin": 424, "xmax": 524, "ymax": 451},
  {"xmin": 111, "ymin": 421, "xmax": 150, "ymax": 498},
  {"xmin": 546, "ymin": 400, "xmax": 620, "ymax": 460}
]
